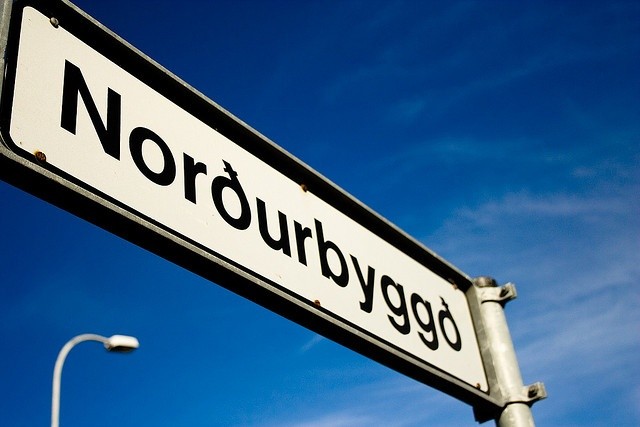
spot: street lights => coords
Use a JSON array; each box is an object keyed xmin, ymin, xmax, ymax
[{"xmin": 51, "ymin": 333, "xmax": 139, "ymax": 427}]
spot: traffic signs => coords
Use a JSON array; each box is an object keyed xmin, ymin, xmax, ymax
[{"xmin": 0, "ymin": 0, "xmax": 505, "ymax": 411}]
